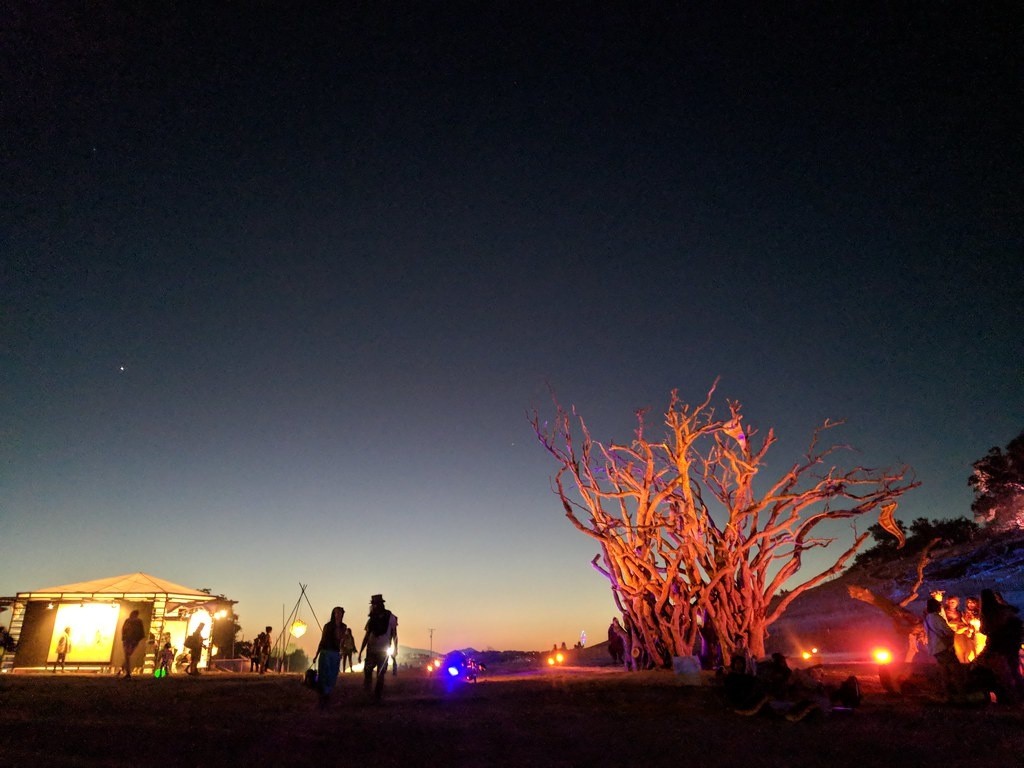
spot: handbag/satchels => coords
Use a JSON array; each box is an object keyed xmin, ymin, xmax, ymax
[{"xmin": 303, "ymin": 664, "xmax": 318, "ymax": 690}]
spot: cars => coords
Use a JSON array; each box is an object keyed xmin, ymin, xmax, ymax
[{"xmin": 789, "ymin": 603, "xmax": 905, "ymax": 694}]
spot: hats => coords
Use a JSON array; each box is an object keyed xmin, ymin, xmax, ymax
[{"xmin": 369, "ymin": 594, "xmax": 385, "ymax": 604}]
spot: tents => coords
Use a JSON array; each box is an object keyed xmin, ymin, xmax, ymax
[{"xmin": 0, "ymin": 573, "xmax": 219, "ymax": 673}]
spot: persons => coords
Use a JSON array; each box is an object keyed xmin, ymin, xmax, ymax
[
  {"xmin": 608, "ymin": 617, "xmax": 624, "ymax": 667},
  {"xmin": 358, "ymin": 595, "xmax": 398, "ymax": 700},
  {"xmin": 185, "ymin": 623, "xmax": 206, "ymax": 676},
  {"xmin": 971, "ymin": 589, "xmax": 1024, "ymax": 692},
  {"xmin": 710, "ymin": 635, "xmax": 765, "ymax": 710},
  {"xmin": 52, "ymin": 627, "xmax": 72, "ymax": 673},
  {"xmin": 314, "ymin": 607, "xmax": 347, "ymax": 711},
  {"xmin": 763, "ymin": 652, "xmax": 793, "ymax": 702},
  {"xmin": 250, "ymin": 627, "xmax": 272, "ymax": 674},
  {"xmin": 157, "ymin": 632, "xmax": 174, "ymax": 679},
  {"xmin": 341, "ymin": 628, "xmax": 357, "ymax": 674},
  {"xmin": 922, "ymin": 599, "xmax": 959, "ymax": 698},
  {"xmin": 115, "ymin": 610, "xmax": 145, "ymax": 681}
]
